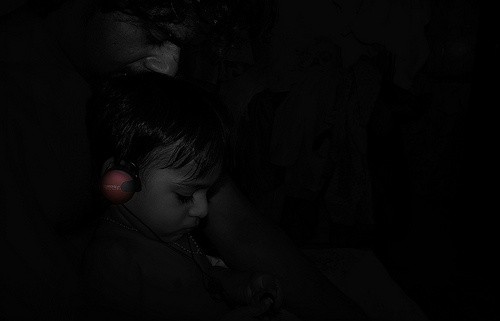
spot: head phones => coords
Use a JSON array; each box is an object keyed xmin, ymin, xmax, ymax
[{"xmin": 96, "ymin": 152, "xmax": 141, "ymax": 206}]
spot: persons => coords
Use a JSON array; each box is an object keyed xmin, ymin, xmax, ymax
[
  {"xmin": 0, "ymin": 0, "xmax": 372, "ymax": 321},
  {"xmin": 79, "ymin": 70, "xmax": 293, "ymax": 321}
]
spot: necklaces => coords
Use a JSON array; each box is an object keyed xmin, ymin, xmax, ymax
[{"xmin": 101, "ymin": 217, "xmax": 203, "ymax": 259}]
